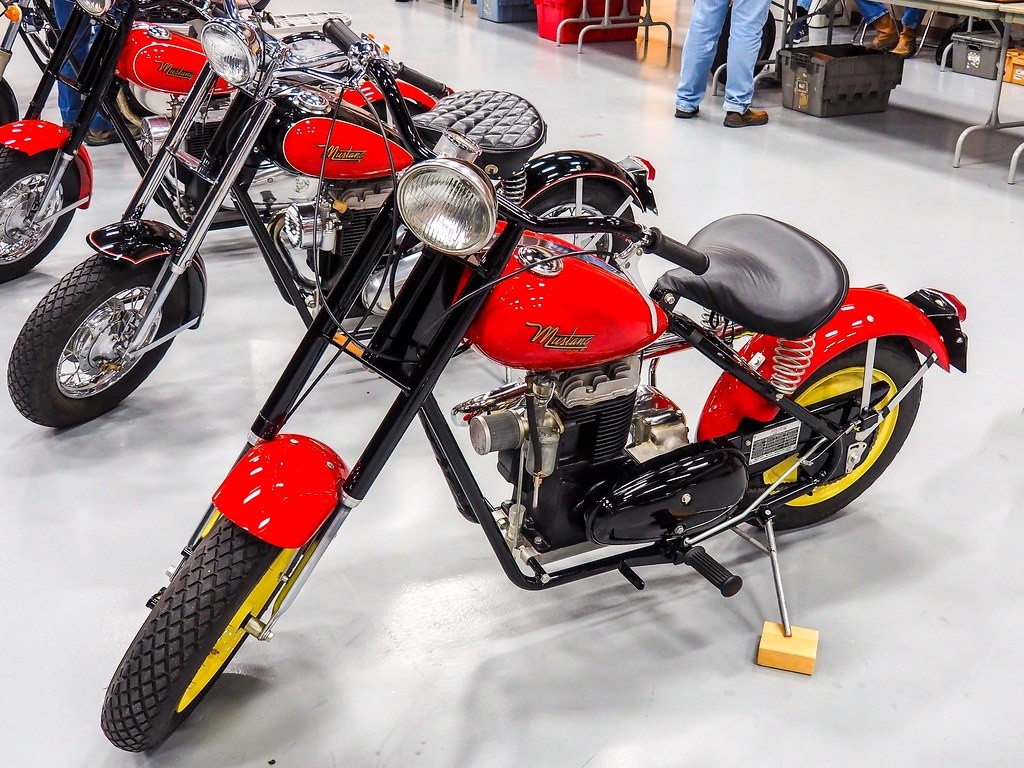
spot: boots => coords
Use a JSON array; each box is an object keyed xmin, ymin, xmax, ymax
[
  {"xmin": 891, "ymin": 25, "xmax": 917, "ymax": 59},
  {"xmin": 865, "ymin": 13, "xmax": 899, "ymax": 52}
]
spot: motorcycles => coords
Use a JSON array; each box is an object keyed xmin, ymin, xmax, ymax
[
  {"xmin": 1, "ymin": 0, "xmax": 665, "ymax": 430},
  {"xmin": 97, "ymin": 25, "xmax": 970, "ymax": 752}
]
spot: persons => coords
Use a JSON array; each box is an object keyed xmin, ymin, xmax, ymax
[
  {"xmin": 854, "ymin": 0, "xmax": 927, "ymax": 59},
  {"xmin": 785, "ymin": 0, "xmax": 812, "ymax": 44},
  {"xmin": 53, "ymin": 0, "xmax": 142, "ymax": 146},
  {"xmin": 674, "ymin": 0, "xmax": 770, "ymax": 128}
]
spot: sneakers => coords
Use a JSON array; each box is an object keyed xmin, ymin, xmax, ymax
[{"xmin": 792, "ymin": 24, "xmax": 809, "ymax": 44}]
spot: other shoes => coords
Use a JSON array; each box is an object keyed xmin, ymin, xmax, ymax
[
  {"xmin": 675, "ymin": 104, "xmax": 700, "ymax": 119},
  {"xmin": 724, "ymin": 107, "xmax": 769, "ymax": 128}
]
